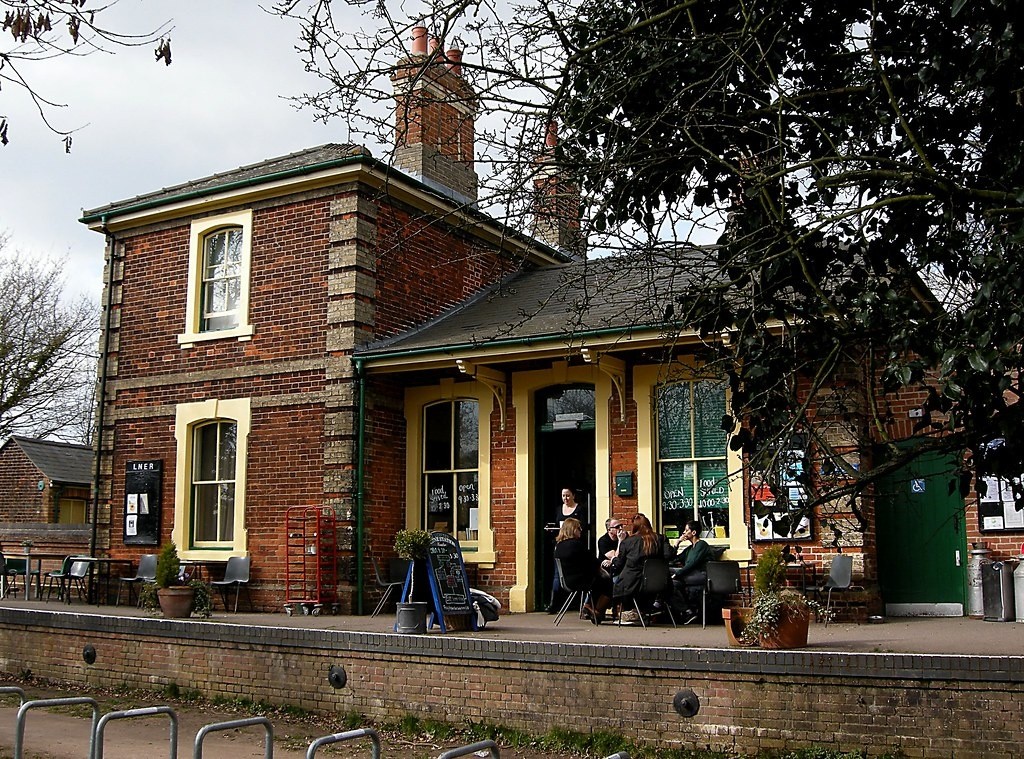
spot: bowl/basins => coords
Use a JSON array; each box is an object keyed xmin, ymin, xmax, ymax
[{"xmin": 869, "ymin": 615, "xmax": 885, "ymax": 624}]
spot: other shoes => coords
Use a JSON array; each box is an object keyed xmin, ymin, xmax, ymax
[
  {"xmin": 591, "ymin": 617, "xmax": 601, "ymax": 624},
  {"xmin": 547, "ymin": 606, "xmax": 554, "ymax": 614},
  {"xmin": 584, "ymin": 604, "xmax": 599, "ymax": 615}
]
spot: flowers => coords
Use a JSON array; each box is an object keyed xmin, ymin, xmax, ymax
[
  {"xmin": 752, "ymin": 587, "xmax": 840, "ymax": 638},
  {"xmin": 18, "ymin": 539, "xmax": 35, "ymax": 547}
]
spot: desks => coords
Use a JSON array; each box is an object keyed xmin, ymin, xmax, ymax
[
  {"xmin": 0, "ymin": 551, "xmax": 69, "ymax": 601},
  {"xmin": 69, "ymin": 557, "xmax": 136, "ymax": 608},
  {"xmin": 748, "ymin": 561, "xmax": 820, "ymax": 623},
  {"xmin": 157, "ymin": 559, "xmax": 229, "ymax": 613}
]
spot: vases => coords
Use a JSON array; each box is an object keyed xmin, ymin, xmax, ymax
[
  {"xmin": 759, "ymin": 603, "xmax": 810, "ymax": 649},
  {"xmin": 23, "ymin": 546, "xmax": 31, "ymax": 553}
]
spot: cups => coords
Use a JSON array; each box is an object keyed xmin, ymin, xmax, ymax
[
  {"xmin": 547, "ymin": 523, "xmax": 555, "ymax": 528},
  {"xmin": 559, "ymin": 521, "xmax": 565, "ymax": 528}
]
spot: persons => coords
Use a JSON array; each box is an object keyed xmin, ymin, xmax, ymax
[{"xmin": 544, "ymin": 485, "xmax": 711, "ymax": 625}]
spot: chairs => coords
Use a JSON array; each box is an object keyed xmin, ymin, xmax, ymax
[
  {"xmin": 679, "ymin": 547, "xmax": 722, "ymax": 626},
  {"xmin": 370, "ymin": 555, "xmax": 405, "ymax": 618},
  {"xmin": 553, "ymin": 558, "xmax": 598, "ymax": 627},
  {"xmin": 6, "ymin": 558, "xmax": 26, "ymax": 598},
  {"xmin": 46, "ymin": 556, "xmax": 89, "ymax": 605},
  {"xmin": 211, "ymin": 557, "xmax": 254, "ymax": 615},
  {"xmin": 74, "ymin": 554, "xmax": 89, "ymax": 590},
  {"xmin": 702, "ymin": 561, "xmax": 746, "ymax": 630},
  {"xmin": 806, "ymin": 555, "xmax": 860, "ymax": 629},
  {"xmin": 39, "ymin": 556, "xmax": 77, "ymax": 601},
  {"xmin": 136, "ymin": 566, "xmax": 186, "ymax": 609},
  {"xmin": 618, "ymin": 557, "xmax": 677, "ymax": 630},
  {"xmin": 115, "ymin": 554, "xmax": 159, "ymax": 608}
]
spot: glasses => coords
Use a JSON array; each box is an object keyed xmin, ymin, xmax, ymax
[
  {"xmin": 578, "ymin": 527, "xmax": 582, "ymax": 531},
  {"xmin": 610, "ymin": 524, "xmax": 622, "ymax": 529}
]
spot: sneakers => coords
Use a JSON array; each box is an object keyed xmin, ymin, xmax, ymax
[
  {"xmin": 684, "ymin": 612, "xmax": 697, "ymax": 625},
  {"xmin": 646, "ymin": 604, "xmax": 665, "ymax": 616}
]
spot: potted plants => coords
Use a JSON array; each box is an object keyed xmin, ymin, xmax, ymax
[
  {"xmin": 139, "ymin": 541, "xmax": 212, "ymax": 619},
  {"xmin": 392, "ymin": 528, "xmax": 433, "ymax": 635}
]
